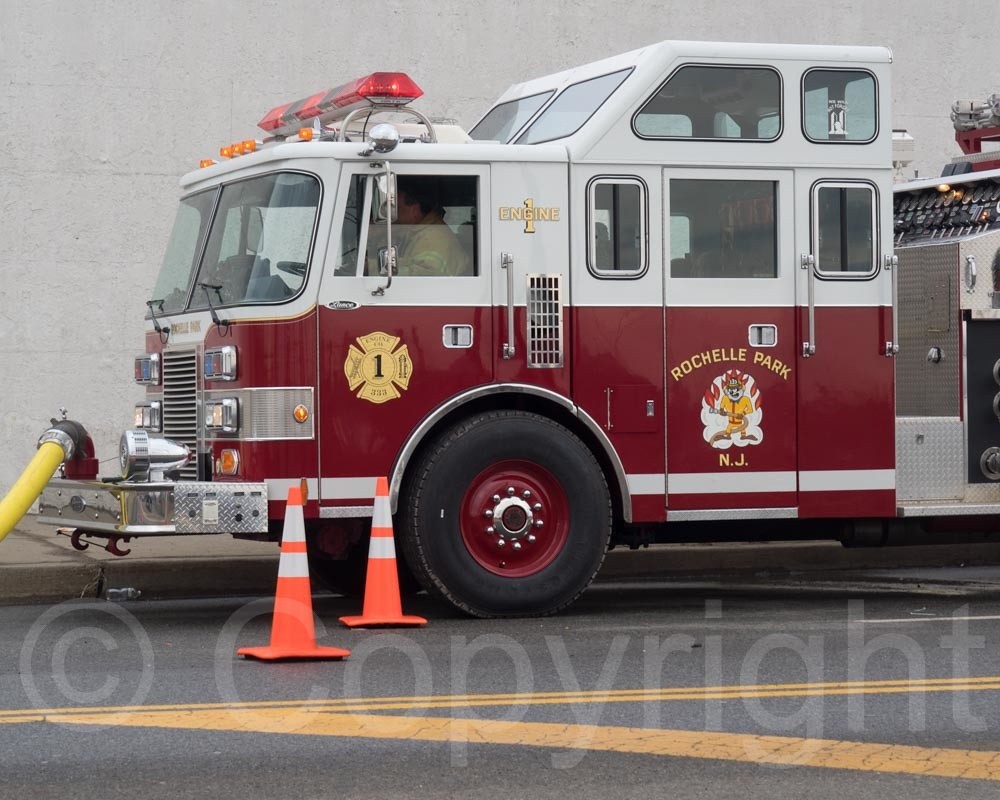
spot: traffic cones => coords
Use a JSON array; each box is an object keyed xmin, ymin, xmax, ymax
[
  {"xmin": 237, "ymin": 486, "xmax": 351, "ymax": 660},
  {"xmin": 338, "ymin": 476, "xmax": 428, "ymax": 626}
]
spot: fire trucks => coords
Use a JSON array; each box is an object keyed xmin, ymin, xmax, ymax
[{"xmin": 38, "ymin": 41, "xmax": 1000, "ymax": 619}]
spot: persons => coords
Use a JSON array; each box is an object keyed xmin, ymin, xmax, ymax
[{"xmin": 335, "ymin": 177, "xmax": 473, "ymax": 276}]
